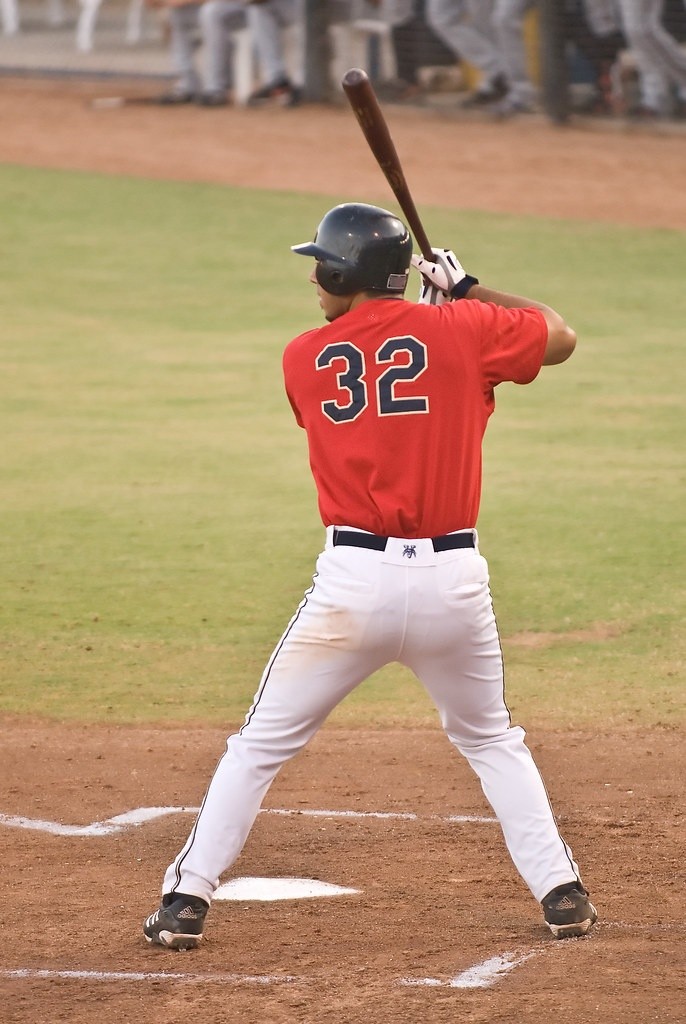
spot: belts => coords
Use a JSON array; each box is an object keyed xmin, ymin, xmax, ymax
[{"xmin": 333, "ymin": 529, "xmax": 475, "ymax": 553}]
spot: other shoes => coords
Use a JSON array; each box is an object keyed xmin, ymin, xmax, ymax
[{"xmin": 136, "ymin": 69, "xmax": 686, "ymax": 131}]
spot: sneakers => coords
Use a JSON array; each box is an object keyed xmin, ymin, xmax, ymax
[
  {"xmin": 142, "ymin": 893, "xmax": 212, "ymax": 951},
  {"xmin": 540, "ymin": 882, "xmax": 598, "ymax": 939}
]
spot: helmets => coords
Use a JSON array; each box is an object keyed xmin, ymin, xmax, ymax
[{"xmin": 290, "ymin": 202, "xmax": 413, "ymax": 296}]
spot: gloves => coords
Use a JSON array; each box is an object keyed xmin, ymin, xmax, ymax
[
  {"xmin": 417, "ymin": 276, "xmax": 448, "ymax": 306},
  {"xmin": 411, "ymin": 248, "xmax": 479, "ymax": 300}
]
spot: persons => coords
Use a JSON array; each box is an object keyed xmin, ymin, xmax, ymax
[
  {"xmin": 145, "ymin": 0, "xmax": 686, "ymax": 124},
  {"xmin": 142, "ymin": 203, "xmax": 601, "ymax": 950}
]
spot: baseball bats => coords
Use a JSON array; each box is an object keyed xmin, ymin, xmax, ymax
[{"xmin": 339, "ymin": 65, "xmax": 437, "ymax": 264}]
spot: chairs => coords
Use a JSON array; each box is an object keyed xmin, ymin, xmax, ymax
[{"xmin": 184, "ymin": 1, "xmax": 287, "ymax": 107}]
